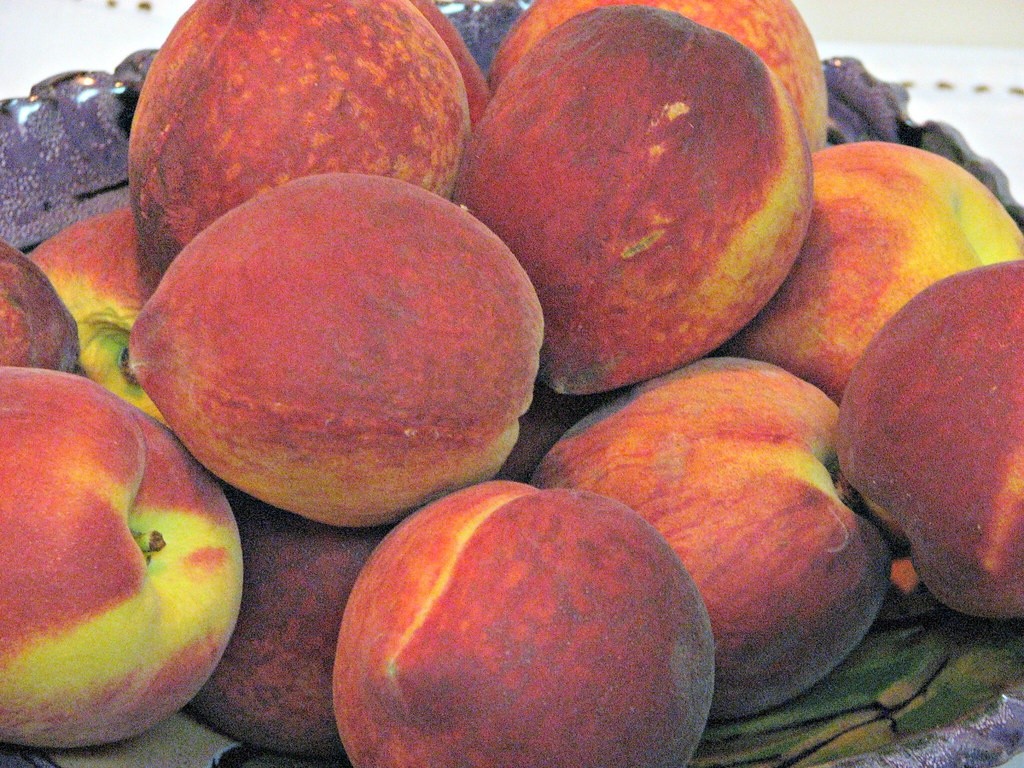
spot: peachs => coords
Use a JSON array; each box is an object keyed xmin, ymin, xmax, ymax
[{"xmin": 0, "ymin": 0, "xmax": 1024, "ymax": 768}]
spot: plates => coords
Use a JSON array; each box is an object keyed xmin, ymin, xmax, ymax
[{"xmin": 0, "ymin": 12, "xmax": 1022, "ymax": 767}]
